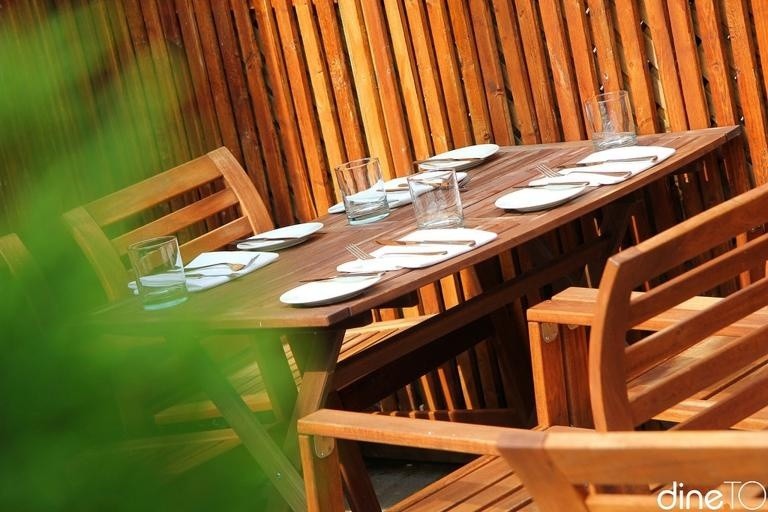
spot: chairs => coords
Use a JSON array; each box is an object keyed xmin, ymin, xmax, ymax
[{"xmin": 1, "ymin": 143, "xmax": 767, "ymax": 510}]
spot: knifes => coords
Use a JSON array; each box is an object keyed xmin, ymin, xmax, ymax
[
  {"xmin": 411, "ymin": 157, "xmax": 486, "ymax": 166},
  {"xmin": 298, "ymin": 272, "xmax": 379, "ymax": 282},
  {"xmin": 236, "ymin": 237, "xmax": 299, "ymax": 243},
  {"xmin": 185, "ymin": 274, "xmax": 241, "ymax": 279},
  {"xmin": 556, "ymin": 155, "xmax": 658, "ymax": 169},
  {"xmin": 512, "ymin": 182, "xmax": 590, "ymax": 189},
  {"xmin": 375, "ymin": 239, "xmax": 476, "ymax": 247}
]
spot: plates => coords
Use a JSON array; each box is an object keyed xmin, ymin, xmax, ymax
[
  {"xmin": 416, "ymin": 143, "xmax": 500, "ymax": 173},
  {"xmin": 237, "ymin": 222, "xmax": 325, "ymax": 252},
  {"xmin": 494, "ymin": 180, "xmax": 586, "ymax": 213},
  {"xmin": 279, "ymin": 271, "xmax": 382, "ymax": 308}
]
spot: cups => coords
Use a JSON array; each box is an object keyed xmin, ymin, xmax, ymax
[
  {"xmin": 584, "ymin": 90, "xmax": 639, "ymax": 153},
  {"xmin": 406, "ymin": 167, "xmax": 464, "ymax": 230},
  {"xmin": 127, "ymin": 236, "xmax": 190, "ymax": 312},
  {"xmin": 333, "ymin": 156, "xmax": 391, "ymax": 226}
]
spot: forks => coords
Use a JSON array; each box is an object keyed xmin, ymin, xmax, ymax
[
  {"xmin": 184, "ymin": 253, "xmax": 260, "ymax": 272},
  {"xmin": 398, "ymin": 173, "xmax": 474, "ymax": 189},
  {"xmin": 344, "ymin": 241, "xmax": 448, "ymax": 261},
  {"xmin": 534, "ymin": 163, "xmax": 632, "ymax": 179}
]
